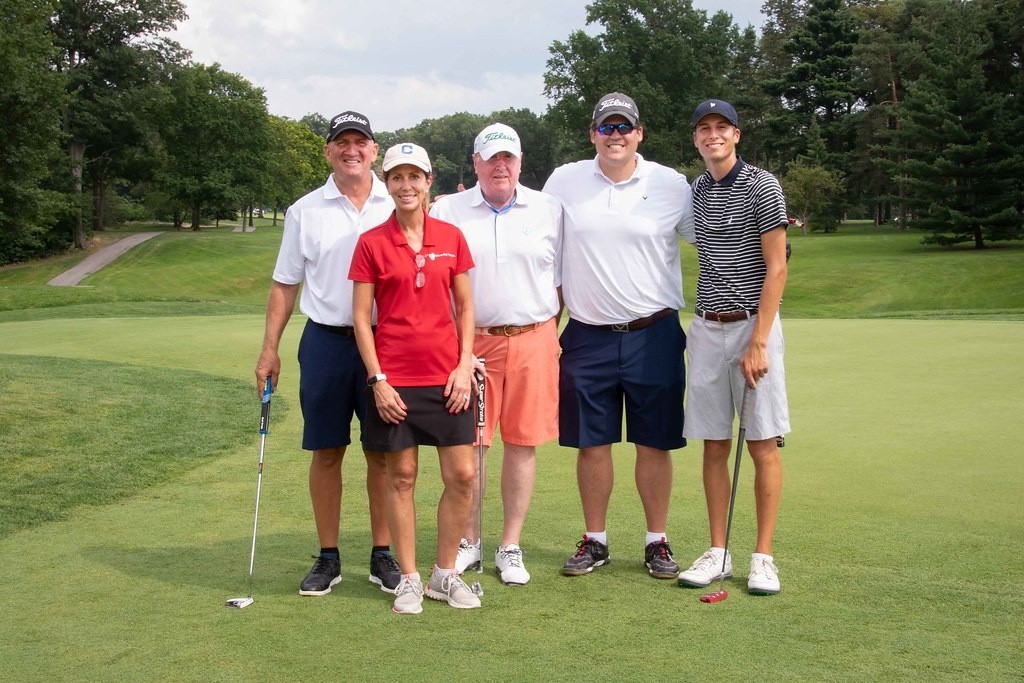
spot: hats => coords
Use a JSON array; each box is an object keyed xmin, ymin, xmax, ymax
[
  {"xmin": 325, "ymin": 110, "xmax": 376, "ymax": 142},
  {"xmin": 474, "ymin": 123, "xmax": 521, "ymax": 162},
  {"xmin": 692, "ymin": 99, "xmax": 738, "ymax": 129},
  {"xmin": 382, "ymin": 143, "xmax": 432, "ymax": 179},
  {"xmin": 591, "ymin": 91, "xmax": 640, "ymax": 128}
]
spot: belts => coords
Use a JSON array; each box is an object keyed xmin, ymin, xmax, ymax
[
  {"xmin": 313, "ymin": 321, "xmax": 355, "ymax": 338},
  {"xmin": 695, "ymin": 305, "xmax": 758, "ymax": 324},
  {"xmin": 474, "ymin": 323, "xmax": 545, "ymax": 337},
  {"xmin": 609, "ymin": 306, "xmax": 672, "ymax": 332}
]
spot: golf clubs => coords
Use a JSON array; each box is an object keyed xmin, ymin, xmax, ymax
[
  {"xmin": 224, "ymin": 376, "xmax": 271, "ymax": 609},
  {"xmin": 699, "ymin": 377, "xmax": 752, "ymax": 604},
  {"xmin": 470, "ymin": 355, "xmax": 486, "ymax": 597}
]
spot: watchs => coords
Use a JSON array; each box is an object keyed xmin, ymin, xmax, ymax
[{"xmin": 367, "ymin": 374, "xmax": 387, "ymax": 386}]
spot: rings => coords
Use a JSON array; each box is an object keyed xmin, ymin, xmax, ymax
[{"xmin": 465, "ymin": 395, "xmax": 468, "ymax": 400}]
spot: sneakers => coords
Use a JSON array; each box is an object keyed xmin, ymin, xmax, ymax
[
  {"xmin": 677, "ymin": 551, "xmax": 733, "ymax": 588},
  {"xmin": 746, "ymin": 558, "xmax": 781, "ymax": 595},
  {"xmin": 298, "ymin": 551, "xmax": 342, "ymax": 596},
  {"xmin": 424, "ymin": 571, "xmax": 482, "ymax": 609},
  {"xmin": 494, "ymin": 544, "xmax": 530, "ymax": 586},
  {"xmin": 643, "ymin": 536, "xmax": 680, "ymax": 579},
  {"xmin": 392, "ymin": 572, "xmax": 424, "ymax": 614},
  {"xmin": 368, "ymin": 552, "xmax": 402, "ymax": 595},
  {"xmin": 454, "ymin": 538, "xmax": 483, "ymax": 575},
  {"xmin": 561, "ymin": 534, "xmax": 610, "ymax": 576}
]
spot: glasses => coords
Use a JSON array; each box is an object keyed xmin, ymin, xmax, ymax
[
  {"xmin": 596, "ymin": 124, "xmax": 635, "ymax": 136},
  {"xmin": 415, "ymin": 253, "xmax": 429, "ymax": 288}
]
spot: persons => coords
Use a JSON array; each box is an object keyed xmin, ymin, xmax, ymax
[
  {"xmin": 256, "ymin": 111, "xmax": 404, "ymax": 599},
  {"xmin": 427, "ymin": 90, "xmax": 695, "ymax": 577},
  {"xmin": 429, "ymin": 120, "xmax": 566, "ymax": 588},
  {"xmin": 349, "ymin": 142, "xmax": 481, "ymax": 616},
  {"xmin": 676, "ymin": 99, "xmax": 789, "ymax": 594}
]
